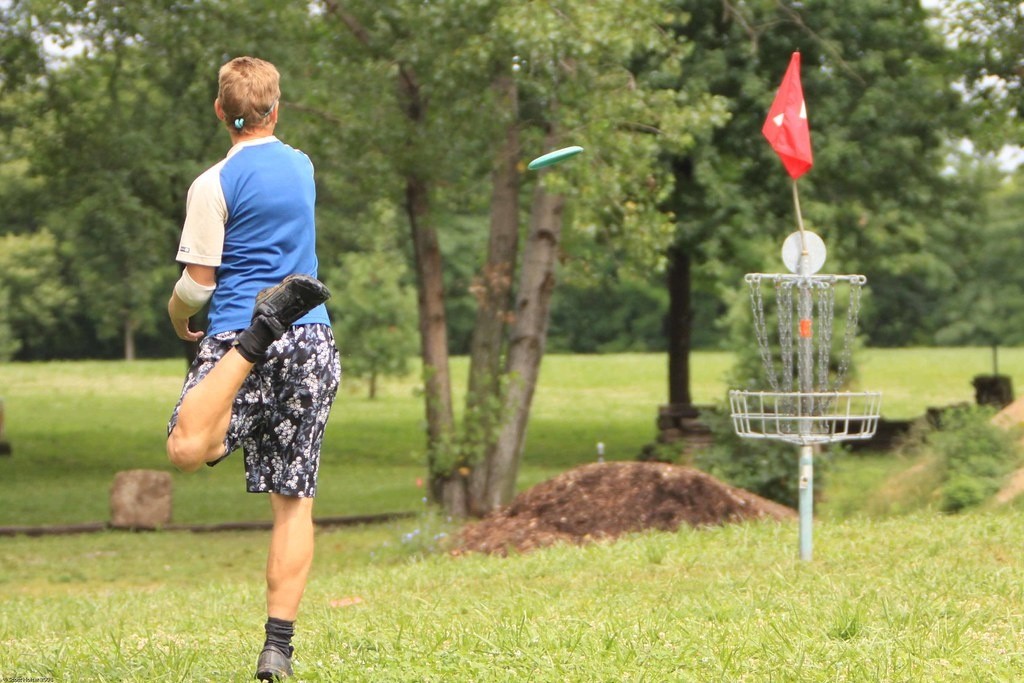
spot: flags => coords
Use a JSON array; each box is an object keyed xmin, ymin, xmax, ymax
[{"xmin": 759, "ymin": 51, "xmax": 814, "ymax": 181}]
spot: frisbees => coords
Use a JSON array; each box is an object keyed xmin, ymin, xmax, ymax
[{"xmin": 527, "ymin": 146, "xmax": 584, "ymax": 170}]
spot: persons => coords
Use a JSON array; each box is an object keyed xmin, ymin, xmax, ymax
[
  {"xmin": 0, "ymin": 402, "xmax": 12, "ymax": 456},
  {"xmin": 166, "ymin": 56, "xmax": 345, "ymax": 683}
]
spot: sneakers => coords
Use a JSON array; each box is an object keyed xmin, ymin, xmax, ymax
[
  {"xmin": 257, "ymin": 647, "xmax": 294, "ymax": 683},
  {"xmin": 251, "ymin": 274, "xmax": 330, "ymax": 341}
]
